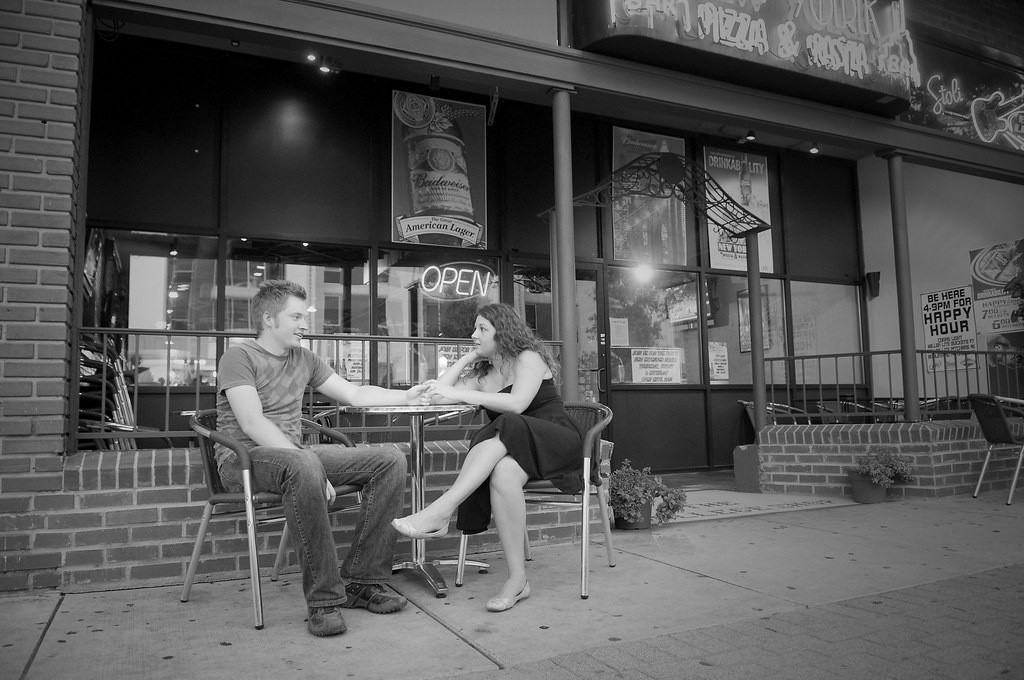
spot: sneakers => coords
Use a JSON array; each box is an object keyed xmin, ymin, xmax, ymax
[
  {"xmin": 338, "ymin": 583, "xmax": 408, "ymax": 614},
  {"xmin": 306, "ymin": 601, "xmax": 346, "ymax": 636}
]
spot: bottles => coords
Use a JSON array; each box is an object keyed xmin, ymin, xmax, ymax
[
  {"xmin": 739, "ymin": 156, "xmax": 753, "ymax": 204},
  {"xmin": 401, "ymin": 93, "xmax": 476, "ymax": 249}
]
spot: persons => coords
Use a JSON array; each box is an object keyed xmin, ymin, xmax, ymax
[
  {"xmin": 390, "ymin": 305, "xmax": 584, "ymax": 612},
  {"xmin": 215, "ymin": 278, "xmax": 431, "ymax": 636}
]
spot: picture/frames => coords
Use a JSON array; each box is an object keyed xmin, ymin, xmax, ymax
[{"xmin": 736, "ymin": 283, "xmax": 772, "ymax": 353}]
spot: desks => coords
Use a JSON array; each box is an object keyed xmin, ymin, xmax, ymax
[{"xmin": 343, "ymin": 402, "xmax": 491, "ymax": 599}]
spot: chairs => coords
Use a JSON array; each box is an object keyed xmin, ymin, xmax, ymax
[
  {"xmin": 817, "ymin": 399, "xmax": 878, "ymax": 425},
  {"xmin": 871, "ymin": 395, "xmax": 971, "ymax": 423},
  {"xmin": 178, "ymin": 408, "xmax": 365, "ymax": 631},
  {"xmin": 455, "ymin": 400, "xmax": 616, "ymax": 600},
  {"xmin": 737, "ymin": 398, "xmax": 812, "ymax": 446},
  {"xmin": 70, "ymin": 336, "xmax": 176, "ymax": 452},
  {"xmin": 967, "ymin": 393, "xmax": 1024, "ymax": 505}
]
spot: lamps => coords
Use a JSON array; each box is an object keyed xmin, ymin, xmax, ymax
[
  {"xmin": 746, "ymin": 130, "xmax": 756, "ymax": 141},
  {"xmin": 809, "ymin": 142, "xmax": 820, "ymax": 154},
  {"xmin": 866, "ymin": 271, "xmax": 881, "ymax": 298}
]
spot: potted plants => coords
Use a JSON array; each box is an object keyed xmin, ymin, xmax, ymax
[
  {"xmin": 845, "ymin": 446, "xmax": 916, "ymax": 505},
  {"xmin": 606, "ymin": 457, "xmax": 687, "ymax": 530}
]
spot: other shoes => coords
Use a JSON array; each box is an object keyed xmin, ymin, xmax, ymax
[
  {"xmin": 391, "ymin": 513, "xmax": 451, "ymax": 539},
  {"xmin": 487, "ymin": 580, "xmax": 530, "ymax": 611}
]
